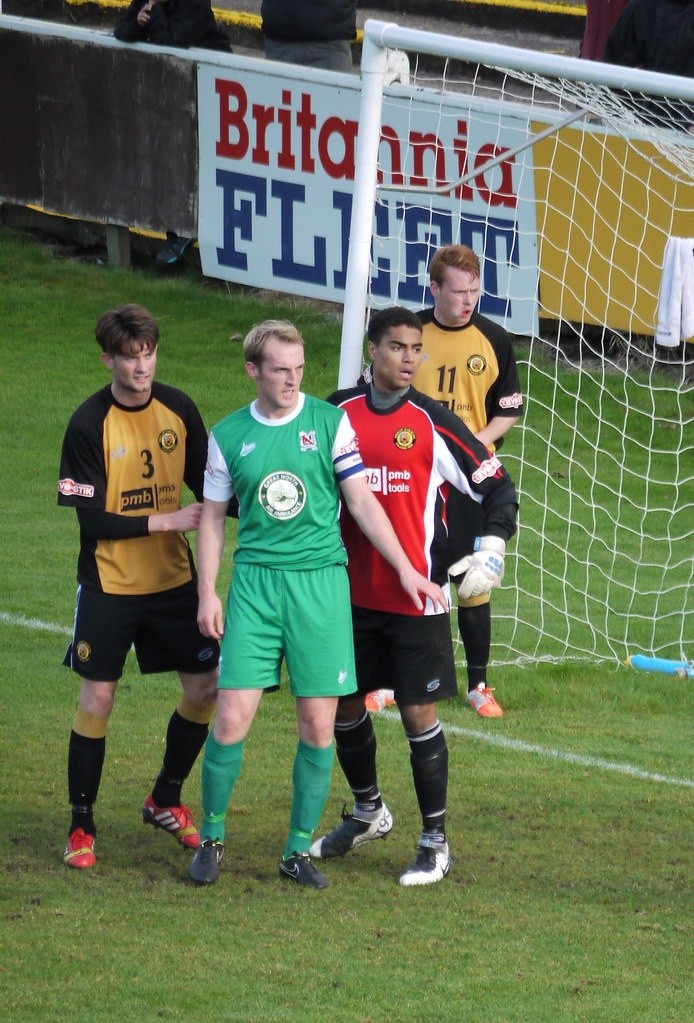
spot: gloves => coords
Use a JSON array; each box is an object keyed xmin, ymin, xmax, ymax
[{"xmin": 445, "ymin": 537, "xmax": 506, "ymax": 599}]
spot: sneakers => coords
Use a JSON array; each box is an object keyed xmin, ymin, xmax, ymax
[
  {"xmin": 188, "ymin": 838, "xmax": 225, "ymax": 884},
  {"xmin": 278, "ymin": 853, "xmax": 330, "ymax": 890},
  {"xmin": 465, "ymin": 681, "xmax": 503, "ymax": 719},
  {"xmin": 63, "ymin": 825, "xmax": 98, "ymax": 868},
  {"xmin": 310, "ymin": 804, "xmax": 394, "ymax": 859},
  {"xmin": 365, "ymin": 689, "xmax": 396, "ymax": 712},
  {"xmin": 398, "ymin": 829, "xmax": 451, "ymax": 886},
  {"xmin": 156, "ymin": 238, "xmax": 193, "ymax": 263},
  {"xmin": 142, "ymin": 794, "xmax": 201, "ymax": 850}
]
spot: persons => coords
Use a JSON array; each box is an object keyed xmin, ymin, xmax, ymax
[
  {"xmin": 185, "ymin": 319, "xmax": 450, "ymax": 892},
  {"xmin": 307, "ymin": 306, "xmax": 520, "ymax": 887},
  {"xmin": 580, "ymin": 1, "xmax": 694, "ymax": 133},
  {"xmin": 112, "ymin": 0, "xmax": 235, "ymax": 266},
  {"xmin": 365, "ymin": 243, "xmax": 524, "ymax": 718},
  {"xmin": 56, "ymin": 306, "xmax": 239, "ymax": 867},
  {"xmin": 259, "ymin": 0, "xmax": 357, "ymax": 74}
]
